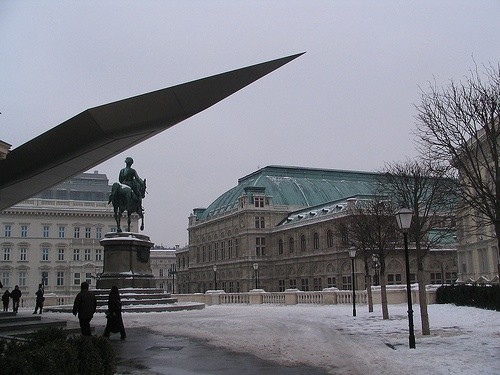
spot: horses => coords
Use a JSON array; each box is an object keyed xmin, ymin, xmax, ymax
[{"xmin": 108, "ymin": 179, "xmax": 147, "ymax": 232}]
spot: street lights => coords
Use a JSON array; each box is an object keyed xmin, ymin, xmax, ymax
[
  {"xmin": 348, "ymin": 243, "xmax": 360, "ymax": 316},
  {"xmin": 253, "ymin": 261, "xmax": 259, "ymax": 289},
  {"xmin": 393, "ymin": 200, "xmax": 419, "ymax": 350},
  {"xmin": 213, "ymin": 263, "xmax": 217, "ymax": 290},
  {"xmin": 372, "ymin": 251, "xmax": 378, "ymax": 286},
  {"xmin": 171, "ymin": 263, "xmax": 176, "ymax": 293}
]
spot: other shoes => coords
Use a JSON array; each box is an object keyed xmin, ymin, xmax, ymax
[
  {"xmin": 33, "ymin": 312, "xmax": 37, "ymax": 314},
  {"xmin": 121, "ymin": 336, "xmax": 126, "ymax": 339},
  {"xmin": 39, "ymin": 312, "xmax": 42, "ymax": 314}
]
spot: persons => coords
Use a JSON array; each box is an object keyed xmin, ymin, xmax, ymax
[
  {"xmin": 103, "ymin": 286, "xmax": 126, "ymax": 340},
  {"xmin": 2, "ymin": 290, "xmax": 11, "ymax": 311},
  {"xmin": 10, "ymin": 285, "xmax": 21, "ymax": 313},
  {"xmin": 33, "ymin": 289, "xmax": 43, "ymax": 314},
  {"xmin": 119, "ymin": 157, "xmax": 141, "ymax": 213},
  {"xmin": 72, "ymin": 282, "xmax": 97, "ymax": 336}
]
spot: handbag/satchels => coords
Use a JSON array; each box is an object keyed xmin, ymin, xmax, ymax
[{"xmin": 108, "ymin": 294, "xmax": 121, "ymax": 311}]
